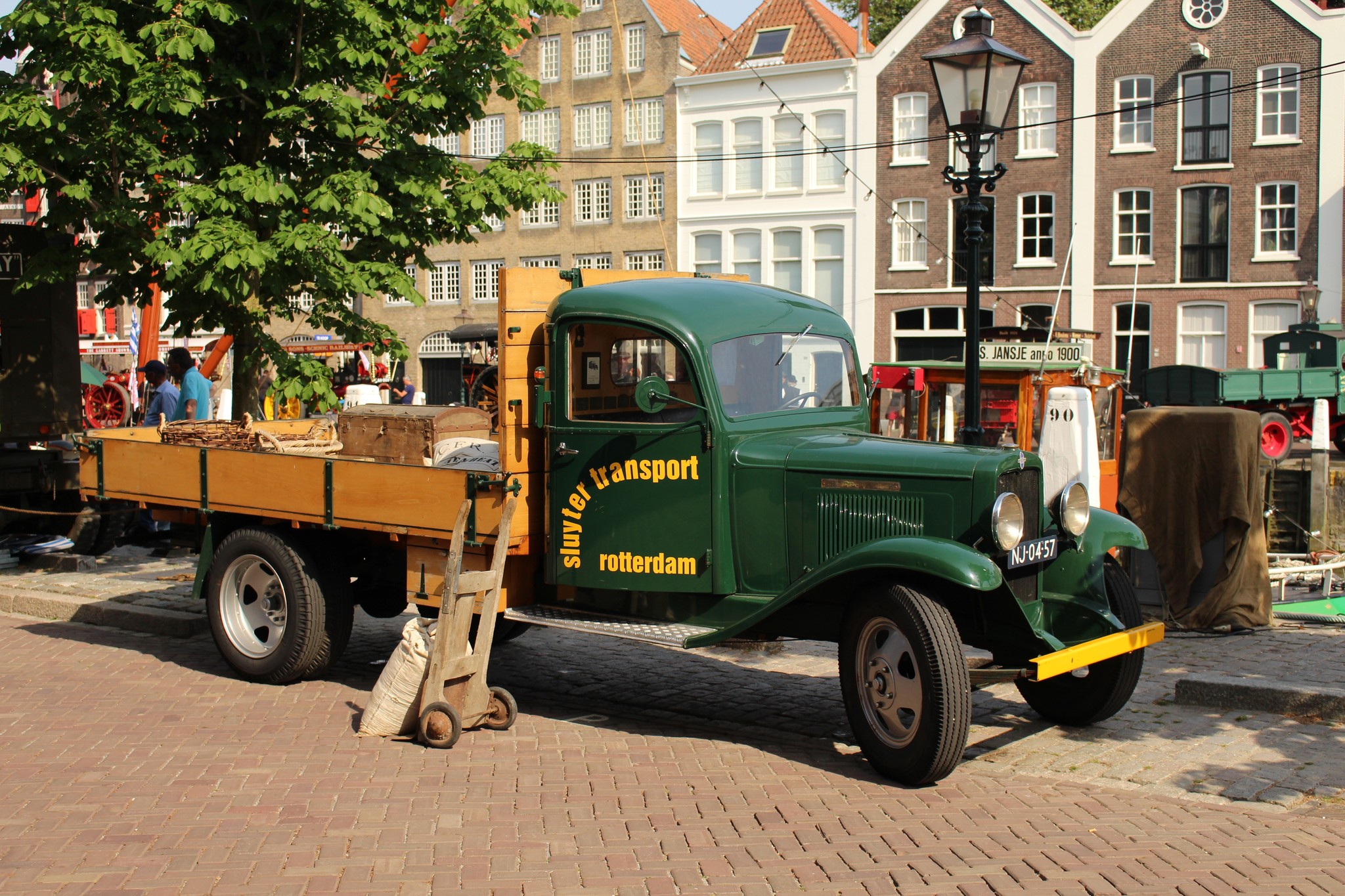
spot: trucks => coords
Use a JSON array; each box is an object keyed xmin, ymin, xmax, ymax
[
  {"xmin": 74, "ymin": 262, "xmax": 1165, "ymax": 789},
  {"xmin": 1141, "ymin": 319, "xmax": 1345, "ymax": 466}
]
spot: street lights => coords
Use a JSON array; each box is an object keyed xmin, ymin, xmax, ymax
[
  {"xmin": 1298, "ymin": 277, "xmax": 1323, "ymax": 323},
  {"xmin": 925, "ymin": 0, "xmax": 1035, "ymax": 453}
]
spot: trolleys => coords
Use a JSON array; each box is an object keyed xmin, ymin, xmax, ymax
[{"xmin": 414, "ymin": 509, "xmax": 518, "ymax": 748}]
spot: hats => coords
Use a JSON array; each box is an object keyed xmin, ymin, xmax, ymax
[{"xmin": 137, "ymin": 360, "xmax": 167, "ymax": 373}]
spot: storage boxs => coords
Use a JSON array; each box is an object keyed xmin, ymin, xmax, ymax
[
  {"xmin": 335, "ymin": 404, "xmax": 493, "ymax": 466},
  {"xmin": 156, "ymin": 411, "xmax": 253, "ymax": 450},
  {"xmin": 250, "ymin": 428, "xmax": 344, "ymax": 459}
]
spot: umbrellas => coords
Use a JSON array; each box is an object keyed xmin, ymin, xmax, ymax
[{"xmin": 81, "ymin": 360, "xmax": 109, "ymax": 388}]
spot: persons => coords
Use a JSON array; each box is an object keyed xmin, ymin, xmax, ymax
[
  {"xmin": 166, "ymin": 347, "xmax": 218, "ymax": 423},
  {"xmin": 134, "ymin": 359, "xmax": 181, "ymax": 535},
  {"xmin": 259, "ymin": 370, "xmax": 276, "ymax": 406},
  {"xmin": 782, "ymin": 371, "xmax": 804, "ymax": 407},
  {"xmin": 393, "ymin": 375, "xmax": 415, "ymax": 405}
]
